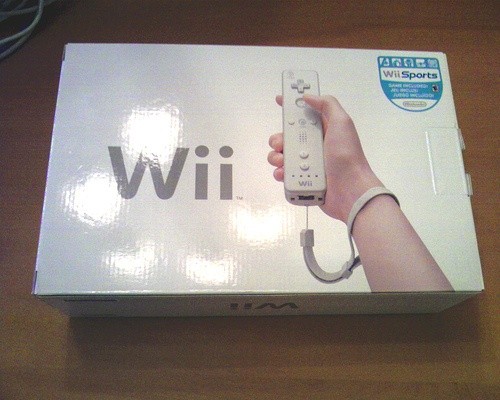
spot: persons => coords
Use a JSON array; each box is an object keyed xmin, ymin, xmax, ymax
[{"xmin": 267, "ymin": 94, "xmax": 454, "ymax": 293}]
